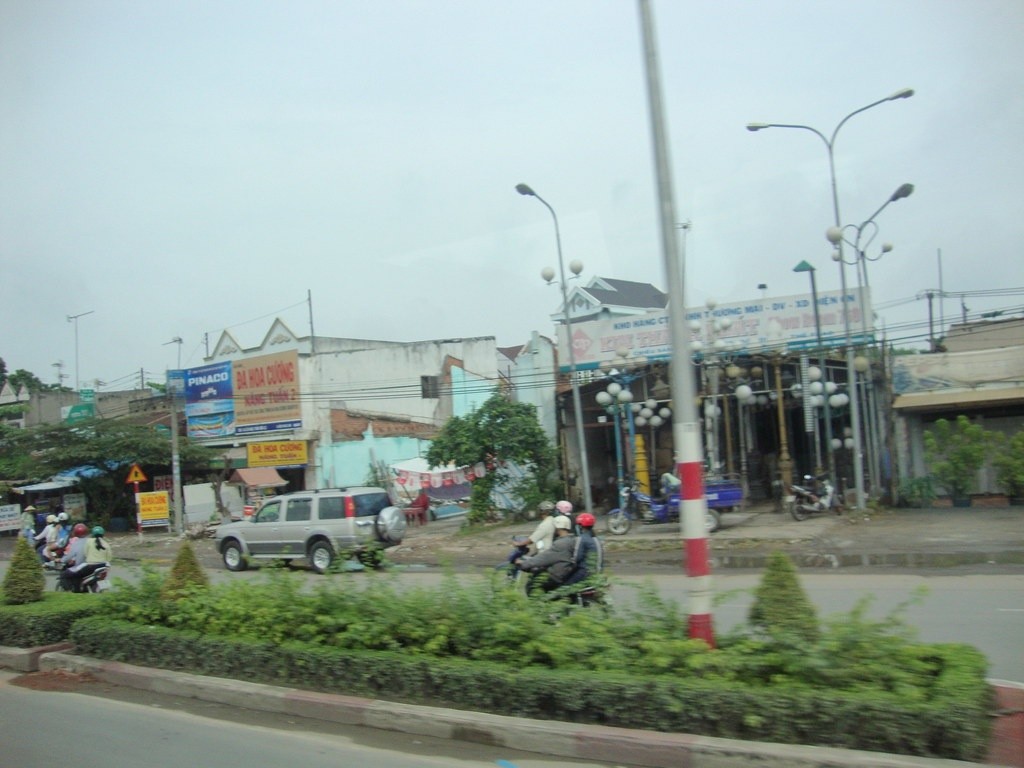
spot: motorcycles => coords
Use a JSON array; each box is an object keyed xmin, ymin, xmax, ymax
[
  {"xmin": 786, "ymin": 469, "xmax": 844, "ymax": 522},
  {"xmin": 55, "ymin": 557, "xmax": 109, "ymax": 593},
  {"xmin": 607, "ymin": 479, "xmax": 744, "ymax": 535},
  {"xmin": 30, "ymin": 528, "xmax": 70, "ymax": 571},
  {"xmin": 491, "ymin": 535, "xmax": 611, "ymax": 620}
]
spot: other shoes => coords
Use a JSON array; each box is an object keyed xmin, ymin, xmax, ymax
[{"xmin": 42, "ymin": 561, "xmax": 49, "ymax": 567}]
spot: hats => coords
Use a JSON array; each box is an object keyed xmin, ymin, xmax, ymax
[{"xmin": 24, "ymin": 505, "xmax": 37, "ymax": 511}]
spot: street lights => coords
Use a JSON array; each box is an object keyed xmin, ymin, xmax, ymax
[
  {"xmin": 692, "ymin": 297, "xmax": 732, "ymax": 471},
  {"xmin": 631, "ymin": 399, "xmax": 672, "ymax": 474},
  {"xmin": 794, "ymin": 261, "xmax": 841, "ymax": 505},
  {"xmin": 855, "ymin": 184, "xmax": 914, "ymax": 491},
  {"xmin": 791, "ymin": 366, "xmax": 837, "ymax": 473},
  {"xmin": 826, "ymin": 220, "xmax": 894, "ymax": 492},
  {"xmin": 745, "ymin": 87, "xmax": 915, "ymax": 511},
  {"xmin": 599, "ymin": 344, "xmax": 648, "ymax": 486},
  {"xmin": 515, "ymin": 183, "xmax": 593, "ymax": 513},
  {"xmin": 596, "ymin": 383, "xmax": 633, "ymax": 508}
]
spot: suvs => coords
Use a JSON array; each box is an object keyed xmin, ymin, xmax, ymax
[{"xmin": 214, "ymin": 486, "xmax": 407, "ymax": 575}]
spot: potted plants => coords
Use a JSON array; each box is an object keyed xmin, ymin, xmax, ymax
[
  {"xmin": 989, "ymin": 428, "xmax": 1024, "ymax": 506},
  {"xmin": 921, "ymin": 415, "xmax": 996, "ymax": 507}
]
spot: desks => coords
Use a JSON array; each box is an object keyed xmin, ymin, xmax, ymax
[{"xmin": 401, "ymin": 508, "xmax": 425, "ymax": 528}]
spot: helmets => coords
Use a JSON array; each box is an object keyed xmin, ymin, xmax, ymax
[
  {"xmin": 92, "ymin": 526, "xmax": 106, "ymax": 537},
  {"xmin": 537, "ymin": 501, "xmax": 553, "ymax": 510},
  {"xmin": 73, "ymin": 523, "xmax": 89, "ymax": 538},
  {"xmin": 555, "ymin": 500, "xmax": 573, "ymax": 514},
  {"xmin": 552, "ymin": 515, "xmax": 572, "ymax": 530},
  {"xmin": 46, "ymin": 515, "xmax": 57, "ymax": 523},
  {"xmin": 59, "ymin": 512, "xmax": 68, "ymax": 521},
  {"xmin": 576, "ymin": 512, "xmax": 596, "ymax": 527}
]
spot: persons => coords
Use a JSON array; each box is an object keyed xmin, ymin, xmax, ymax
[
  {"xmin": 33, "ymin": 513, "xmax": 69, "ymax": 567},
  {"xmin": 413, "ymin": 489, "xmax": 429, "ymax": 524},
  {"xmin": 513, "ymin": 500, "xmax": 604, "ymax": 601},
  {"xmin": 61, "ymin": 524, "xmax": 112, "ymax": 593},
  {"xmin": 21, "ymin": 506, "xmax": 37, "ymax": 546}
]
